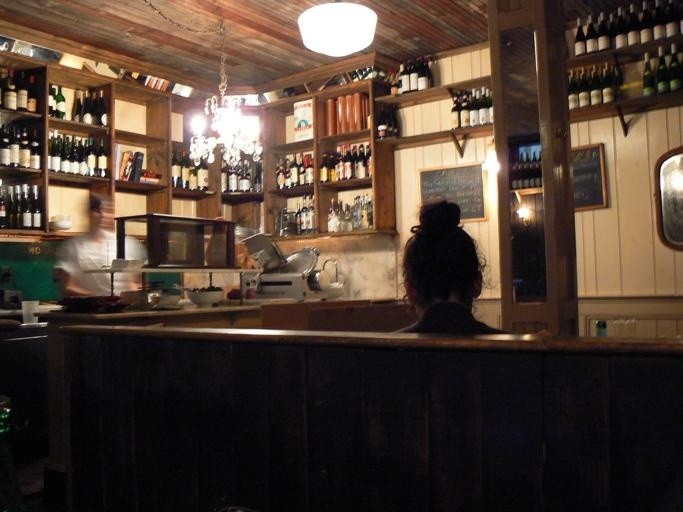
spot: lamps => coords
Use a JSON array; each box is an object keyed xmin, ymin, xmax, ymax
[{"xmin": 297, "ymin": 0, "xmax": 378, "ymax": 57}]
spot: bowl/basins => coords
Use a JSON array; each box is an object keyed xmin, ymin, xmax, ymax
[
  {"xmin": 316, "ymin": 287, "xmax": 345, "ymax": 301},
  {"xmin": 111, "ymin": 259, "xmax": 143, "ymax": 269},
  {"xmin": 186, "ymin": 291, "xmax": 225, "ymax": 308},
  {"xmin": 148, "ymin": 294, "xmax": 180, "ymax": 306},
  {"xmin": 49, "ymin": 221, "xmax": 73, "ymax": 232}
]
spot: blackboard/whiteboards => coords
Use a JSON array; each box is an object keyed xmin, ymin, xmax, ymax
[
  {"xmin": 572, "ymin": 143, "xmax": 607, "ymax": 212},
  {"xmin": 417, "ymin": 161, "xmax": 488, "ymax": 223}
]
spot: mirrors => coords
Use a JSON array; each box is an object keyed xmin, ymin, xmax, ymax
[{"xmin": 654, "ymin": 142, "xmax": 683, "ymax": 252}]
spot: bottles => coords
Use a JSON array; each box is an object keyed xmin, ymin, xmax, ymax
[
  {"xmin": 48, "ymin": 81, "xmax": 108, "ymax": 178},
  {"xmin": 451, "ymin": 86, "xmax": 494, "ymax": 130},
  {"xmin": 567, "ymin": 0, "xmax": 683, "ymax": 110},
  {"xmin": 172, "ymin": 148, "xmax": 262, "ymax": 193},
  {"xmin": 0, "ymin": 66, "xmax": 44, "ymax": 230},
  {"xmin": 376, "ymin": 103, "xmax": 400, "ymax": 137},
  {"xmin": 595, "ymin": 319, "xmax": 607, "ymax": 337},
  {"xmin": 390, "ymin": 55, "xmax": 434, "ymax": 97},
  {"xmin": 511, "ymin": 151, "xmax": 542, "ymax": 189},
  {"xmin": 132, "ymin": 152, "xmax": 144, "ymax": 183},
  {"xmin": 272, "ymin": 145, "xmax": 373, "ymax": 238},
  {"xmin": 351, "ymin": 66, "xmax": 372, "ymax": 81}
]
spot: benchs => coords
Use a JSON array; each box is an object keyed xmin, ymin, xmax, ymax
[{"xmin": 60, "ymin": 319, "xmax": 682, "ymax": 512}]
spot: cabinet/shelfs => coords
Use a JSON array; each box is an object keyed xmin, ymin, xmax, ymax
[
  {"xmin": 564, "ymin": 34, "xmax": 682, "ymax": 135},
  {"xmin": 0, "ymin": 51, "xmax": 398, "ymax": 240},
  {"xmin": 374, "ymin": 74, "xmax": 495, "ymax": 156}
]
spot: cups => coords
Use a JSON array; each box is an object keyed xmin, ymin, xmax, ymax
[{"xmin": 22, "ymin": 300, "xmax": 40, "ymax": 324}]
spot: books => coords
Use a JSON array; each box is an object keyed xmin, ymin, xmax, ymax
[
  {"xmin": 114, "ymin": 143, "xmax": 162, "ymax": 184},
  {"xmin": 324, "ymin": 91, "xmax": 367, "ymax": 135},
  {"xmin": 146, "ymin": 74, "xmax": 170, "ymax": 92}
]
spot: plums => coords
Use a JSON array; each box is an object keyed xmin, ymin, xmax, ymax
[{"xmin": 227, "ymin": 288, "xmax": 240, "ymax": 300}]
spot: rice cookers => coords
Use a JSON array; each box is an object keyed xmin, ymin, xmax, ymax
[{"xmin": 257, "ymin": 245, "xmax": 320, "ymax": 303}]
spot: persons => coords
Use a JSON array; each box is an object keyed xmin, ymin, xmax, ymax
[
  {"xmin": 394, "ymin": 199, "xmax": 508, "ymax": 336},
  {"xmin": 52, "ymin": 192, "xmax": 147, "ymax": 297}
]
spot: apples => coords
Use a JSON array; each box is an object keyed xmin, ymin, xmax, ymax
[{"xmin": 246, "ymin": 288, "xmax": 256, "ymax": 299}]
